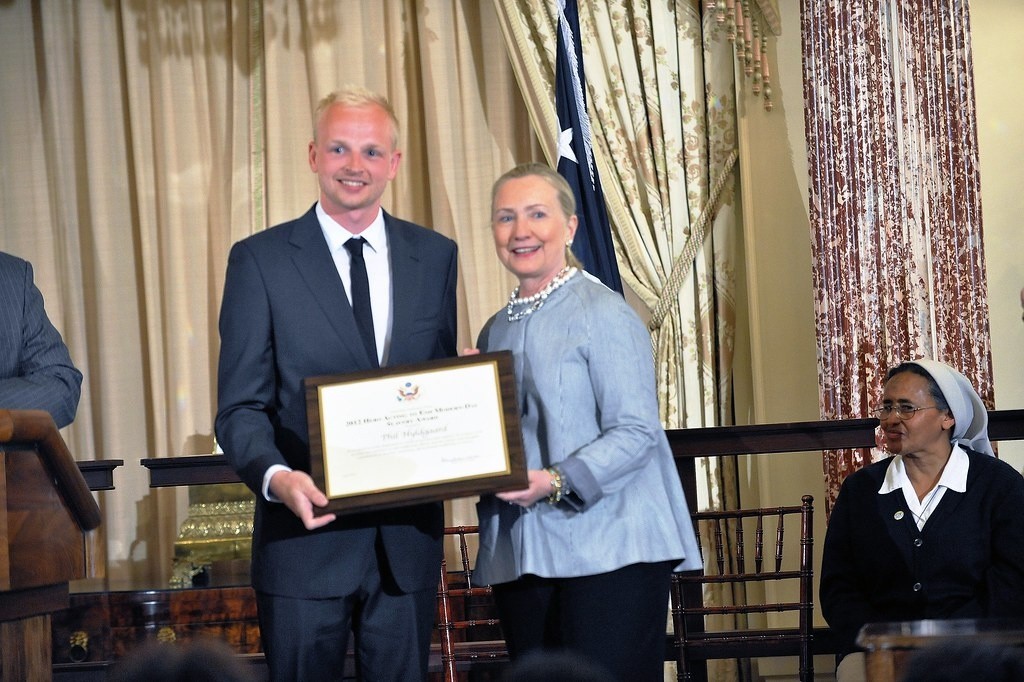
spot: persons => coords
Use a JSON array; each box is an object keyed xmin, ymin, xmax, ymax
[
  {"xmin": 817, "ymin": 357, "xmax": 1024, "ymax": 682},
  {"xmin": 461, "ymin": 160, "xmax": 706, "ymax": 681},
  {"xmin": 208, "ymin": 81, "xmax": 463, "ymax": 681},
  {"xmin": 0, "ymin": 249, "xmax": 84, "ymax": 453}
]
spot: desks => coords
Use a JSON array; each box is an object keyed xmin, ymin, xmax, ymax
[{"xmin": 855, "ymin": 622, "xmax": 1024, "ymax": 682}]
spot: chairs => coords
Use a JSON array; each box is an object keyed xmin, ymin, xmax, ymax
[
  {"xmin": 436, "ymin": 526, "xmax": 510, "ymax": 681},
  {"xmin": 674, "ymin": 494, "xmax": 814, "ymax": 682}
]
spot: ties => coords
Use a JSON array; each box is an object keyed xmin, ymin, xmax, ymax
[{"xmin": 343, "ymin": 237, "xmax": 379, "ymax": 370}]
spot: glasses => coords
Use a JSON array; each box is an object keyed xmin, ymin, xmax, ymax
[{"xmin": 873, "ymin": 401, "xmax": 938, "ymax": 420}]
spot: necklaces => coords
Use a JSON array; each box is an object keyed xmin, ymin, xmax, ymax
[{"xmin": 506, "ymin": 262, "xmax": 579, "ymax": 324}]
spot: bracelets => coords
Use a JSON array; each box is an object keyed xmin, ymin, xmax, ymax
[{"xmin": 547, "ymin": 463, "xmax": 571, "ymax": 505}]
